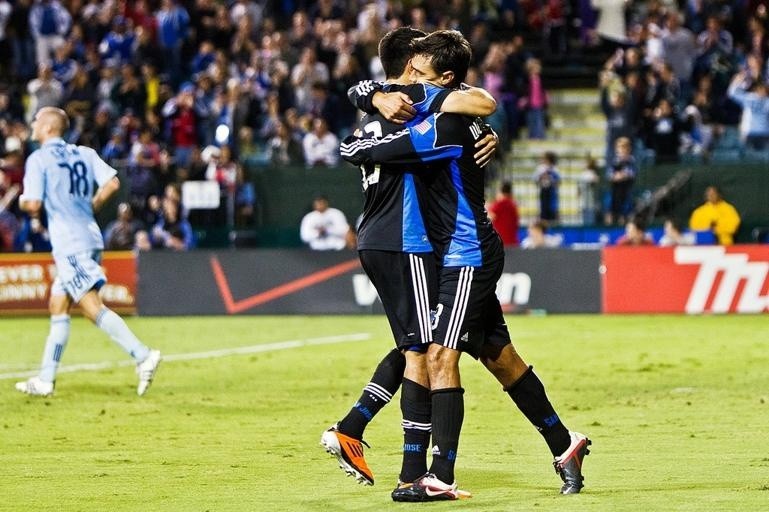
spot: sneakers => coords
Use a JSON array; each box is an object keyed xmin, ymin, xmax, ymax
[
  {"xmin": 14, "ymin": 378, "xmax": 57, "ymax": 398},
  {"xmin": 554, "ymin": 430, "xmax": 592, "ymax": 494},
  {"xmin": 392, "ymin": 473, "xmax": 459, "ymax": 503},
  {"xmin": 397, "ymin": 480, "xmax": 472, "ymax": 500},
  {"xmin": 136, "ymin": 348, "xmax": 161, "ymax": 395},
  {"xmin": 321, "ymin": 422, "xmax": 373, "ymax": 487}
]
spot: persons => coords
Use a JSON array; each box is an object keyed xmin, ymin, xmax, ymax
[
  {"xmin": 336, "ymin": 29, "xmax": 591, "ymax": 495},
  {"xmin": 1, "ymin": 0, "xmax": 769, "ymax": 252},
  {"xmin": 319, "ymin": 30, "xmax": 501, "ymax": 501},
  {"xmin": 13, "ymin": 106, "xmax": 160, "ymax": 397}
]
